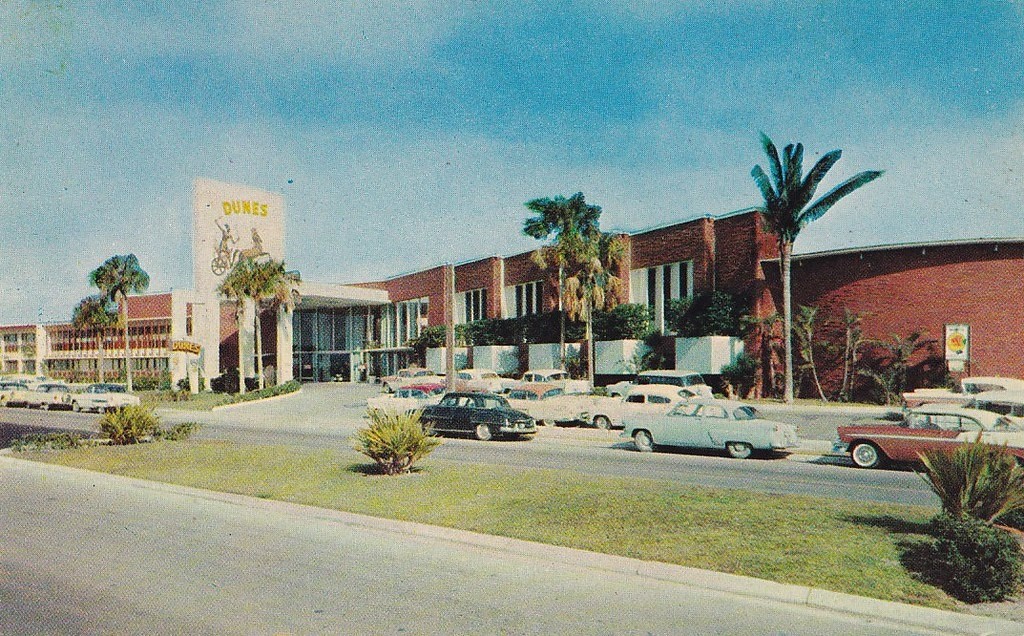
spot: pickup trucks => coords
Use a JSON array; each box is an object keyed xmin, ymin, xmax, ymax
[
  {"xmin": 586, "ymin": 385, "xmax": 696, "ymax": 431},
  {"xmin": 903, "ymin": 377, "xmax": 1023, "ymax": 409}
]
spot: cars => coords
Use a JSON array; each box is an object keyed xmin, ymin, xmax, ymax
[
  {"xmin": 837, "ymin": 408, "xmax": 1024, "ymax": 468},
  {"xmin": 961, "ymin": 390, "xmax": 1024, "ymax": 417},
  {"xmin": 0, "ymin": 381, "xmax": 141, "ymax": 415},
  {"xmin": 403, "ymin": 392, "xmax": 537, "ymax": 442},
  {"xmin": 456, "ymin": 369, "xmax": 503, "ymax": 393},
  {"xmin": 379, "ymin": 369, "xmax": 446, "ymax": 396},
  {"xmin": 619, "ymin": 400, "xmax": 799, "ymax": 460},
  {"xmin": 503, "ymin": 368, "xmax": 588, "ymax": 394},
  {"xmin": 484, "ymin": 383, "xmax": 588, "ymax": 428}
]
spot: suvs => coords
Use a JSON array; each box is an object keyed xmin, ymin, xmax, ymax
[{"xmin": 606, "ymin": 370, "xmax": 709, "ymax": 399}]
